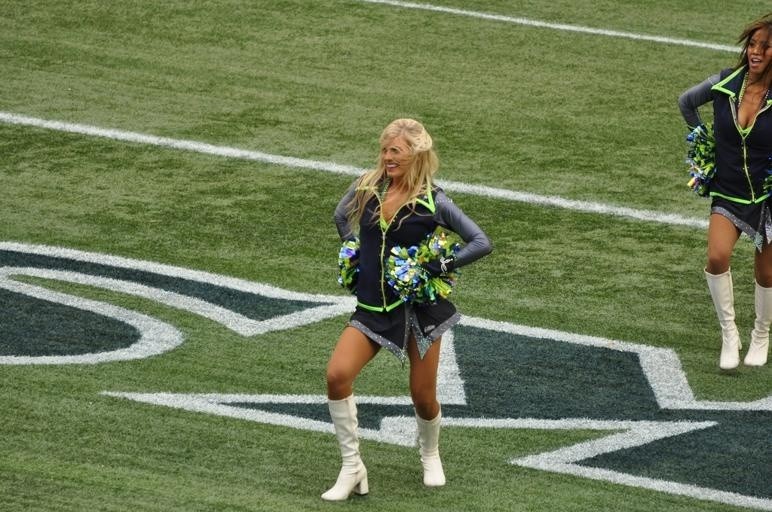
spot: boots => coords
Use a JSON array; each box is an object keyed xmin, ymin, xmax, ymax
[
  {"xmin": 321, "ymin": 394, "xmax": 369, "ymax": 501},
  {"xmin": 704, "ymin": 265, "xmax": 743, "ymax": 369},
  {"xmin": 744, "ymin": 279, "xmax": 772, "ymax": 366},
  {"xmin": 413, "ymin": 401, "xmax": 446, "ymax": 486}
]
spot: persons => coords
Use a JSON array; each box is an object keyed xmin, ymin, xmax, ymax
[
  {"xmin": 678, "ymin": 11, "xmax": 771, "ymax": 371},
  {"xmin": 320, "ymin": 117, "xmax": 494, "ymax": 500}
]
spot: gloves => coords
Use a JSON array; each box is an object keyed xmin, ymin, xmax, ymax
[{"xmin": 423, "ymin": 256, "xmax": 454, "ymax": 276}]
